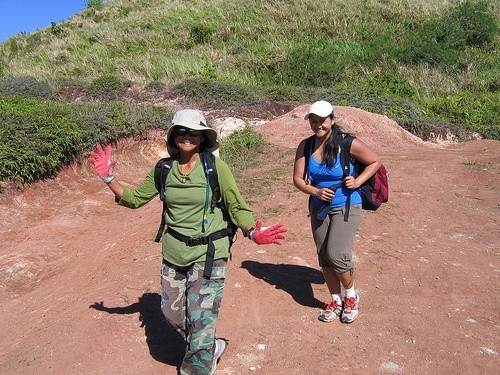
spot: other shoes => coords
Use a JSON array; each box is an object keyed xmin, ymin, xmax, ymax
[{"xmin": 179, "ymin": 337, "xmax": 228, "ymax": 375}]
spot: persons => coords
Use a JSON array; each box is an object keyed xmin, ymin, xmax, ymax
[
  {"xmin": 292, "ymin": 101, "xmax": 382, "ymax": 323},
  {"xmin": 86, "ymin": 109, "xmax": 288, "ymax": 375}
]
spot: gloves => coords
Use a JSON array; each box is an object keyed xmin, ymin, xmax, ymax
[
  {"xmin": 249, "ymin": 219, "xmax": 288, "ymax": 246},
  {"xmin": 87, "ymin": 144, "xmax": 118, "ymax": 184}
]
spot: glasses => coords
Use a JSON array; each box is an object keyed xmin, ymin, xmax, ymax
[{"xmin": 173, "ymin": 127, "xmax": 203, "ymax": 137}]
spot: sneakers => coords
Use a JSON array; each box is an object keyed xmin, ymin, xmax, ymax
[
  {"xmin": 318, "ymin": 300, "xmax": 344, "ymax": 322},
  {"xmin": 341, "ymin": 293, "xmax": 360, "ymax": 323}
]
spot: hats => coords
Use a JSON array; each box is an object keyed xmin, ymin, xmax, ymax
[
  {"xmin": 162, "ymin": 109, "xmax": 219, "ymax": 157},
  {"xmin": 304, "ymin": 100, "xmax": 334, "ymax": 121}
]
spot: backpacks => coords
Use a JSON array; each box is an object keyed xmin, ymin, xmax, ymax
[{"xmin": 304, "ymin": 132, "xmax": 388, "ymax": 221}]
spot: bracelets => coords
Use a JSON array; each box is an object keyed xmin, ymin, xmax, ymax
[{"xmin": 247, "ymin": 226, "xmax": 255, "ymax": 240}]
